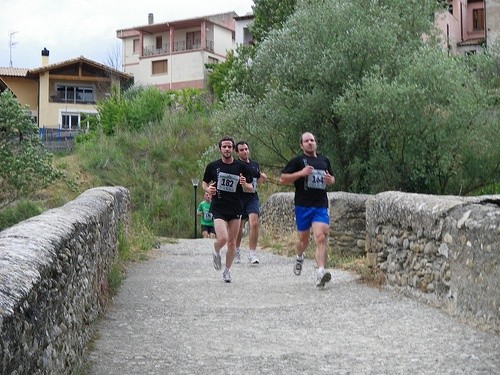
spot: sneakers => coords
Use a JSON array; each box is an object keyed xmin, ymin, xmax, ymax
[
  {"xmin": 213, "ymin": 250, "xmax": 222, "ymax": 270},
  {"xmin": 316, "ymin": 271, "xmax": 331, "ymax": 289},
  {"xmin": 234, "ymin": 252, "xmax": 240, "ymax": 263},
  {"xmin": 223, "ymin": 268, "xmax": 232, "ymax": 282},
  {"xmin": 293, "ymin": 255, "xmax": 304, "ymax": 275},
  {"xmin": 248, "ymin": 255, "xmax": 260, "ymax": 264}
]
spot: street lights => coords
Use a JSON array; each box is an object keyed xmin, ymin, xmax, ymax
[{"xmin": 190, "ymin": 178, "xmax": 200, "ymax": 239}]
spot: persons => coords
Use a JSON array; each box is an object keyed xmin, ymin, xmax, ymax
[
  {"xmin": 202, "ymin": 137, "xmax": 254, "ymax": 282},
  {"xmin": 197, "ymin": 191, "xmax": 215, "ymax": 238},
  {"xmin": 234, "ymin": 140, "xmax": 267, "ymax": 264},
  {"xmin": 280, "ymin": 132, "xmax": 336, "ymax": 287}
]
